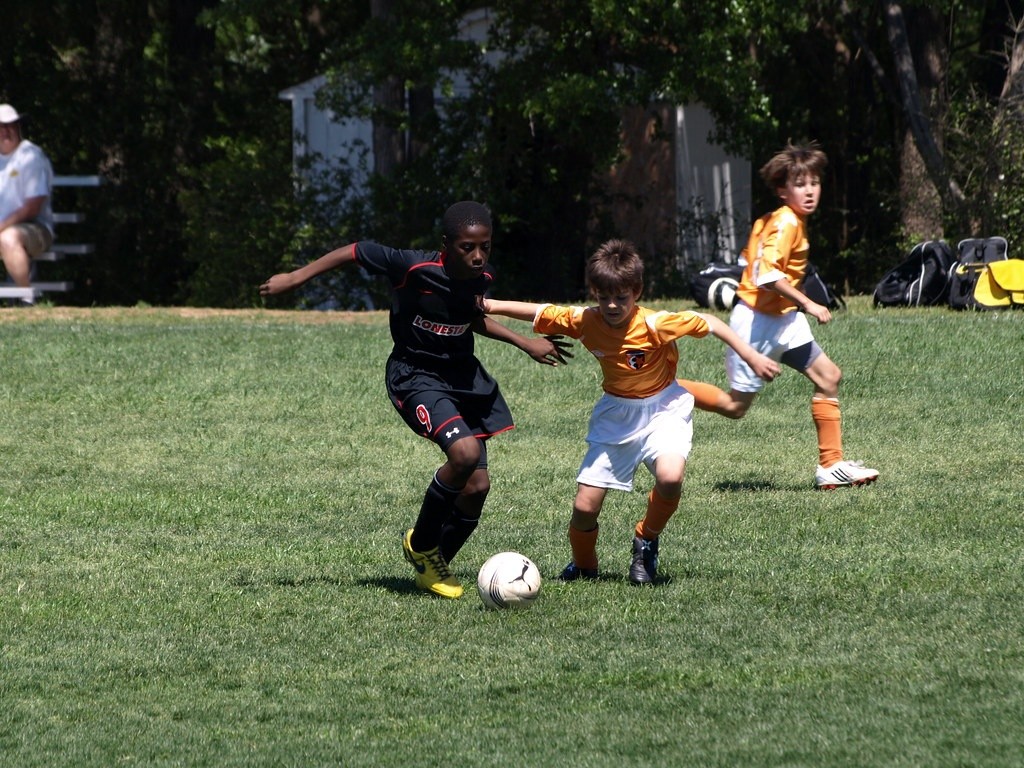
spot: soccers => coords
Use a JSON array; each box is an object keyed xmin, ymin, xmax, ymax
[{"xmin": 478, "ymin": 551, "xmax": 541, "ymax": 610}]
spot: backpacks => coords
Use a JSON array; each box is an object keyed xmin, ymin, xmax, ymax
[
  {"xmin": 872, "ymin": 239, "xmax": 948, "ymax": 308},
  {"xmin": 947, "ymin": 236, "xmax": 1011, "ymax": 309}
]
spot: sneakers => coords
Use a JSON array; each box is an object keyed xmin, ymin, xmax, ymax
[
  {"xmin": 402, "ymin": 529, "xmax": 464, "ymax": 598},
  {"xmin": 630, "ymin": 535, "xmax": 659, "ymax": 583},
  {"xmin": 559, "ymin": 562, "xmax": 598, "ymax": 581},
  {"xmin": 417, "ymin": 571, "xmax": 425, "ymax": 590},
  {"xmin": 816, "ymin": 461, "xmax": 879, "ymax": 490}
]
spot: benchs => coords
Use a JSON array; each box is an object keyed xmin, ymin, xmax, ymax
[{"xmin": 0, "ymin": 175, "xmax": 101, "ymax": 300}]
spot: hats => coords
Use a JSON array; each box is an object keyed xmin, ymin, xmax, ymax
[{"xmin": 0, "ymin": 104, "xmax": 20, "ymax": 123}]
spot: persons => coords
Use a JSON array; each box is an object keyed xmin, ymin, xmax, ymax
[
  {"xmin": 258, "ymin": 202, "xmax": 575, "ymax": 599},
  {"xmin": 0, "ymin": 103, "xmax": 57, "ymax": 308},
  {"xmin": 481, "ymin": 237, "xmax": 783, "ymax": 584},
  {"xmin": 674, "ymin": 140, "xmax": 879, "ymax": 488}
]
